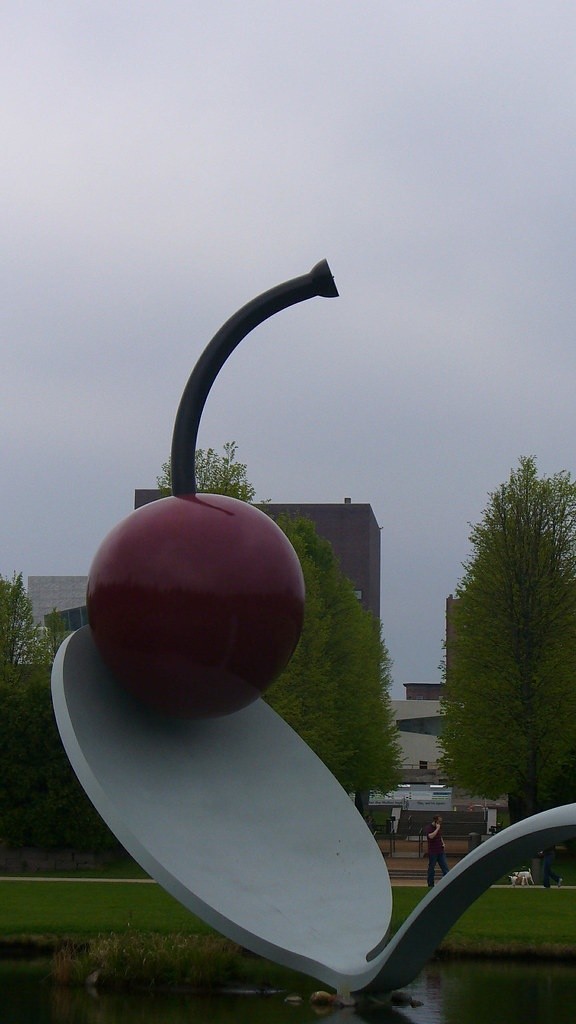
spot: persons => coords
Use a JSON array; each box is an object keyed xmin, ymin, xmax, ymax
[
  {"xmin": 425, "ymin": 815, "xmax": 450, "ymax": 887},
  {"xmin": 540, "ymin": 844, "xmax": 563, "ymax": 888},
  {"xmin": 365, "ymin": 815, "xmax": 378, "ymax": 836}
]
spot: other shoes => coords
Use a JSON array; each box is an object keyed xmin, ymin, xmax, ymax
[{"xmin": 558, "ymin": 878, "xmax": 562, "ymax": 888}]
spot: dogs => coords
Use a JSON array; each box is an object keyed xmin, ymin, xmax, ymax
[
  {"xmin": 507, "ymin": 875, "xmax": 530, "ymax": 887},
  {"xmin": 512, "ymin": 867, "xmax": 535, "ymax": 886}
]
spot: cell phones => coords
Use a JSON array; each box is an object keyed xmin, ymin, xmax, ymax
[{"xmin": 434, "ymin": 821, "xmax": 438, "ymax": 826}]
